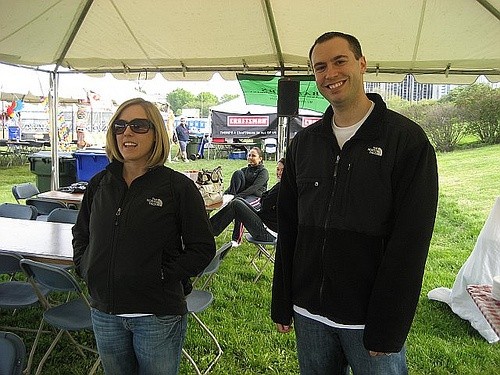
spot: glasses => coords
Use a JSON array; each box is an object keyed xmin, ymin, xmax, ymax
[{"xmin": 112, "ymin": 118, "xmax": 156, "ymax": 135}]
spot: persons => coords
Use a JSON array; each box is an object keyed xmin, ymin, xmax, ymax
[
  {"xmin": 172, "ymin": 117, "xmax": 285, "ymax": 247},
  {"xmin": 71, "ymin": 98, "xmax": 216, "ymax": 375},
  {"xmin": 271, "ymin": 32, "xmax": 439, "ymax": 375}
]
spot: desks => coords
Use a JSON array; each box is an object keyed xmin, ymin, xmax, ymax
[
  {"xmin": 0, "ymin": 217, "xmax": 76, "ymax": 265},
  {"xmin": 6, "ymin": 142, "xmax": 30, "ymax": 167},
  {"xmin": 37, "ymin": 189, "xmax": 233, "ymax": 219},
  {"xmin": 211, "ymin": 142, "xmax": 257, "ymax": 160}
]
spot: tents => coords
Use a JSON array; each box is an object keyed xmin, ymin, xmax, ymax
[{"xmin": 0, "ymin": 1, "xmax": 499, "ymax": 191}]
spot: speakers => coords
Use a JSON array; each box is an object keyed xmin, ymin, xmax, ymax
[{"xmin": 277, "ymin": 78, "xmax": 300, "ymax": 117}]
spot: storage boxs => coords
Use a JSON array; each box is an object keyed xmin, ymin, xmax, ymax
[{"xmin": 231, "ymin": 151, "xmax": 245, "ymax": 159}]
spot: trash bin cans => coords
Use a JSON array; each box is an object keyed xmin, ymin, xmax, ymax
[
  {"xmin": 26, "ymin": 150, "xmax": 77, "ymax": 192},
  {"xmin": 186, "ymin": 135, "xmax": 201, "ymax": 159},
  {"xmin": 72, "ymin": 147, "xmax": 112, "ymax": 182}
]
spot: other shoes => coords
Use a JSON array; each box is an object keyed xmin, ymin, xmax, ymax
[
  {"xmin": 182, "ymin": 160, "xmax": 189, "ymax": 163},
  {"xmin": 231, "ymin": 240, "xmax": 240, "ymax": 248},
  {"xmin": 174, "ymin": 157, "xmax": 179, "ymax": 162}
]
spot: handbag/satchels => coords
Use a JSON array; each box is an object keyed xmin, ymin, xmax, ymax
[{"xmin": 198, "ymin": 183, "xmax": 225, "ymax": 206}]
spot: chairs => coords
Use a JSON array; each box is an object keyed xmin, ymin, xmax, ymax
[
  {"xmin": 264, "ymin": 144, "xmax": 276, "ymax": 162},
  {"xmin": 0, "ymin": 142, "xmax": 44, "ymax": 166},
  {"xmin": 212, "ymin": 138, "xmax": 229, "ymax": 159},
  {"xmin": 0, "ymin": 183, "xmax": 278, "ymax": 375}
]
